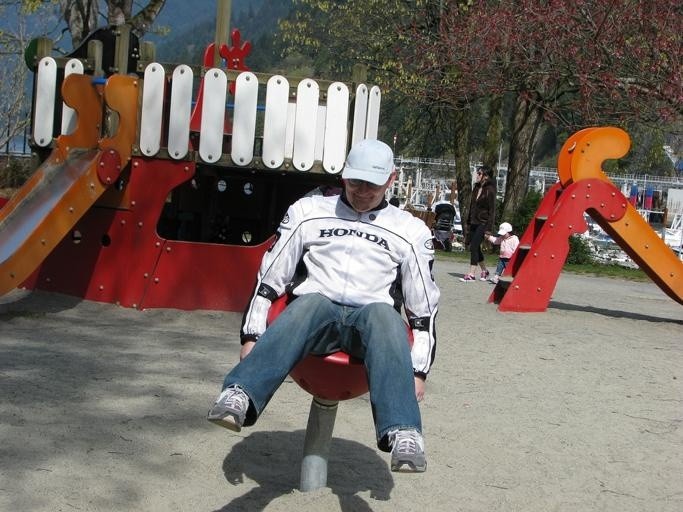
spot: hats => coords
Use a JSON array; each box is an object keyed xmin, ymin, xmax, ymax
[
  {"xmin": 497, "ymin": 222, "xmax": 512, "ymax": 236},
  {"xmin": 342, "ymin": 139, "xmax": 395, "ymax": 186}
]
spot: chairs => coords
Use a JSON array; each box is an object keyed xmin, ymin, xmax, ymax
[{"xmin": 266, "ymin": 294, "xmax": 414, "ymax": 493}]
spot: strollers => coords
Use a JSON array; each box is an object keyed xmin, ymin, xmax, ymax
[{"xmin": 429, "ymin": 203, "xmax": 458, "ymax": 254}]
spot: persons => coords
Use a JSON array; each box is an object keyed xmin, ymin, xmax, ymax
[
  {"xmin": 460, "ymin": 165, "xmax": 497, "ymax": 283},
  {"xmin": 206, "ymin": 140, "xmax": 441, "ymax": 472},
  {"xmin": 484, "ymin": 221, "xmax": 520, "ymax": 284}
]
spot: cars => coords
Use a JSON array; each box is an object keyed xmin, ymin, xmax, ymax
[
  {"xmin": 414, "ymin": 201, "xmax": 463, "ymax": 225},
  {"xmin": 0, "ymin": 131, "xmax": 36, "ymax": 160}
]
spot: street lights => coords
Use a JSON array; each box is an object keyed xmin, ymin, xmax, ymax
[{"xmin": 392, "ymin": 133, "xmax": 397, "ymax": 157}]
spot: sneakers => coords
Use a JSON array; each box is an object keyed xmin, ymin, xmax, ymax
[
  {"xmin": 390, "ymin": 429, "xmax": 427, "ymax": 473},
  {"xmin": 207, "ymin": 387, "xmax": 250, "ymax": 432},
  {"xmin": 489, "ymin": 275, "xmax": 499, "ymax": 284},
  {"xmin": 480, "ymin": 270, "xmax": 489, "ymax": 281},
  {"xmin": 459, "ymin": 274, "xmax": 476, "ymax": 282}
]
